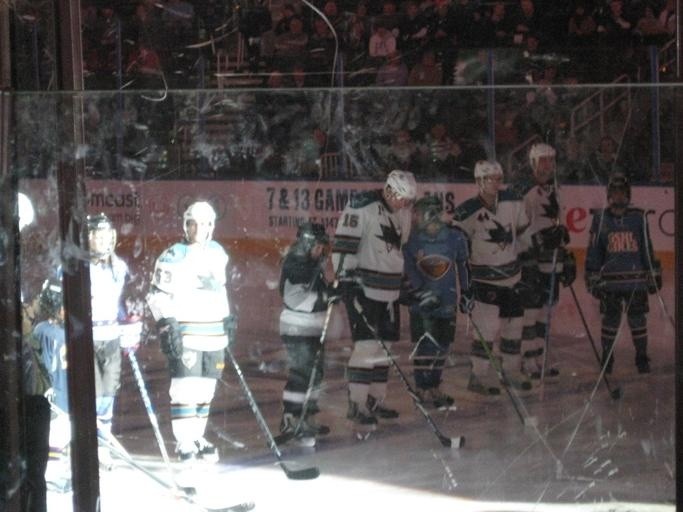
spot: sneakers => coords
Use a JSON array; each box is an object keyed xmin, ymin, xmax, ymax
[
  {"xmin": 601, "ymin": 351, "xmax": 613, "ymax": 372},
  {"xmin": 174, "ymin": 437, "xmax": 218, "ymax": 460},
  {"xmin": 635, "ymin": 352, "xmax": 650, "ymax": 372},
  {"xmin": 415, "ymin": 387, "xmax": 453, "ymax": 408},
  {"xmin": 469, "ymin": 349, "xmax": 559, "ymax": 394}
]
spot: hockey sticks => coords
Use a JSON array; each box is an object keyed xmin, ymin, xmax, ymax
[
  {"xmin": 468, "ymin": 313, "xmax": 540, "ymax": 428},
  {"xmin": 531, "ymin": 247, "xmax": 558, "ymax": 401},
  {"xmin": 49, "ymin": 404, "xmax": 255, "ymax": 511},
  {"xmin": 226, "ymin": 347, "xmax": 321, "ymax": 478},
  {"xmin": 265, "ymin": 280, "xmax": 339, "ymax": 448},
  {"xmin": 127, "ymin": 350, "xmax": 195, "ymax": 495},
  {"xmin": 355, "ymin": 298, "xmax": 464, "ymax": 448},
  {"xmin": 566, "ymin": 278, "xmax": 624, "ymax": 399},
  {"xmin": 643, "ymin": 210, "xmax": 675, "ymax": 327}
]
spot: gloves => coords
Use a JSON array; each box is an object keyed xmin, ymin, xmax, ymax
[
  {"xmin": 584, "ymin": 271, "xmax": 603, "ymax": 298},
  {"xmin": 338, "ymin": 270, "xmax": 368, "ymax": 304},
  {"xmin": 413, "ymin": 288, "xmax": 441, "ymax": 311},
  {"xmin": 648, "ymin": 260, "xmax": 661, "ymax": 293},
  {"xmin": 560, "ymin": 252, "xmax": 576, "ymax": 286},
  {"xmin": 532, "ymin": 225, "xmax": 569, "ymax": 250},
  {"xmin": 321, "ymin": 284, "xmax": 343, "ymax": 306},
  {"xmin": 158, "ymin": 319, "xmax": 181, "ymax": 359},
  {"xmin": 459, "ymin": 290, "xmax": 474, "ymax": 313}
]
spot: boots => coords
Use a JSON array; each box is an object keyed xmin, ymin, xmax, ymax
[
  {"xmin": 346, "ymin": 387, "xmax": 399, "ymax": 425},
  {"xmin": 280, "ymin": 402, "xmax": 330, "ymax": 444}
]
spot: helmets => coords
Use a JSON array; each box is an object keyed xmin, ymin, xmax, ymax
[
  {"xmin": 474, "ymin": 161, "xmax": 505, "ymax": 197},
  {"xmin": 529, "ymin": 143, "xmax": 556, "ymax": 179},
  {"xmin": 606, "ymin": 177, "xmax": 631, "ymax": 207},
  {"xmin": 39, "ymin": 278, "xmax": 65, "ymax": 322},
  {"xmin": 87, "ymin": 213, "xmax": 117, "ymax": 263},
  {"xmin": 182, "ymin": 201, "xmax": 216, "ymax": 245},
  {"xmin": 384, "ymin": 170, "xmax": 418, "ymax": 207},
  {"xmin": 295, "ymin": 222, "xmax": 331, "ymax": 259},
  {"xmin": 414, "ymin": 198, "xmax": 443, "ymax": 230}
]
